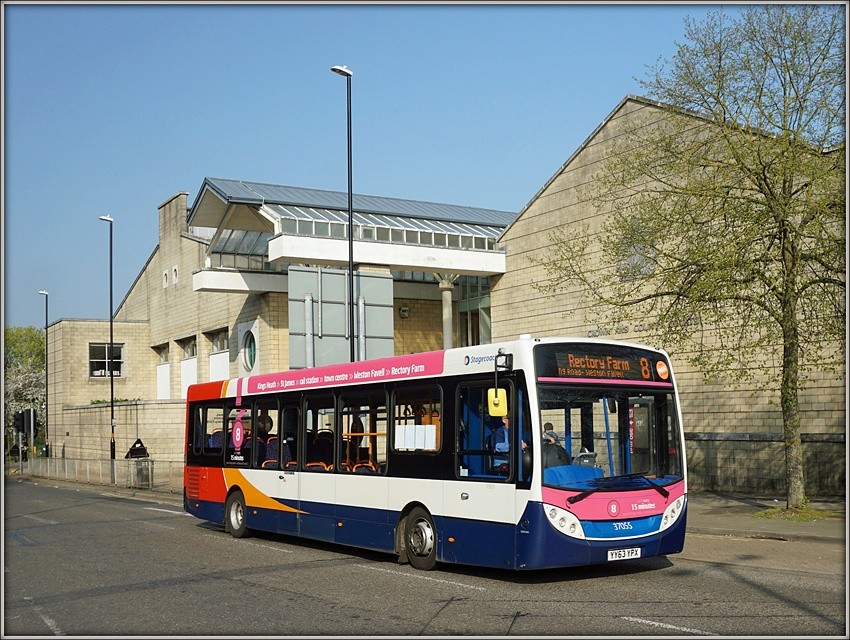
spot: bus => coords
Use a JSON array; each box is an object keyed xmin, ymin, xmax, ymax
[{"xmin": 182, "ymin": 334, "xmax": 688, "ymax": 573}]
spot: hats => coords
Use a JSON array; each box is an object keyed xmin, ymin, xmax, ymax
[{"xmin": 544, "ymin": 423, "xmax": 553, "ymax": 430}]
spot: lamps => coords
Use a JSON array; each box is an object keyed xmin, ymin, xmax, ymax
[{"xmin": 400, "ymin": 306, "xmax": 410, "ymax": 318}]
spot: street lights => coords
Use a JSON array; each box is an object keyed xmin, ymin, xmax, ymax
[
  {"xmin": 99, "ymin": 215, "xmax": 116, "ymax": 483},
  {"xmin": 329, "ymin": 64, "xmax": 357, "ymax": 363},
  {"xmin": 37, "ymin": 290, "xmax": 49, "ymax": 457}
]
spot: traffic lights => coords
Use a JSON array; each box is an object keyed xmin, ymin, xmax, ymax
[{"xmin": 12, "ymin": 413, "xmax": 22, "ymax": 433}]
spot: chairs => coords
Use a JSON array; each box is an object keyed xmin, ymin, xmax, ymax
[{"xmin": 211, "ymin": 428, "xmax": 386, "ymax": 474}]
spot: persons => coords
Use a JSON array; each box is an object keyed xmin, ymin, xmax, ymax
[
  {"xmin": 492, "ymin": 410, "xmax": 527, "ymax": 475},
  {"xmin": 541, "ymin": 422, "xmax": 561, "ymax": 446}
]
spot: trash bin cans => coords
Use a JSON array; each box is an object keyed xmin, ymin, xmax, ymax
[
  {"xmin": 22, "ymin": 450, "xmax": 27, "ymax": 461},
  {"xmin": 135, "ymin": 459, "xmax": 153, "ymax": 489}
]
